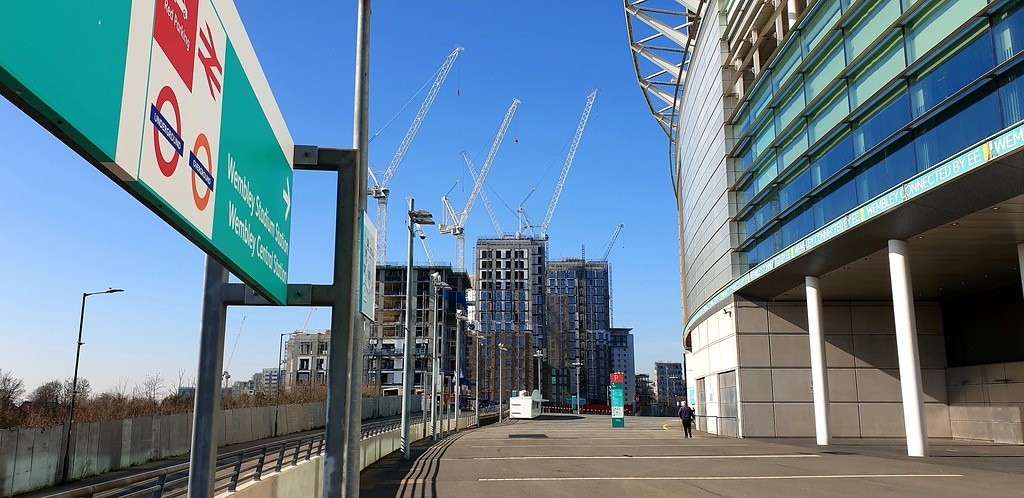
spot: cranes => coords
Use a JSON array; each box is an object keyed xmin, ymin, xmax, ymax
[
  {"xmin": 460, "ymin": 151, "xmax": 542, "ymax": 238},
  {"xmin": 516, "ymin": 87, "xmax": 599, "ymax": 239},
  {"xmin": 222, "ymin": 314, "xmax": 247, "ymax": 388},
  {"xmin": 364, "ymin": 44, "xmax": 466, "ymax": 266},
  {"xmin": 299, "ymin": 307, "xmax": 316, "ymax": 333},
  {"xmin": 601, "ymin": 223, "xmax": 626, "ymax": 262},
  {"xmin": 437, "ymin": 97, "xmax": 521, "ymax": 273},
  {"xmin": 581, "ymin": 244, "xmax": 585, "ymax": 261}
]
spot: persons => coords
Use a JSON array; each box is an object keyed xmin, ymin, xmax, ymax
[{"xmin": 678, "ymin": 401, "xmax": 695, "ymax": 439}]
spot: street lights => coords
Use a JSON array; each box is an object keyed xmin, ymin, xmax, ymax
[
  {"xmin": 533, "ymin": 350, "xmax": 543, "ymax": 398},
  {"xmin": 275, "ymin": 333, "xmax": 300, "ymax": 437},
  {"xmin": 497, "ymin": 343, "xmax": 509, "ymax": 423},
  {"xmin": 572, "ymin": 363, "xmax": 584, "ymax": 414},
  {"xmin": 400, "ymin": 197, "xmax": 436, "ymax": 462},
  {"xmin": 62, "ymin": 287, "xmax": 125, "ymax": 487},
  {"xmin": 453, "ymin": 309, "xmax": 470, "ymax": 433},
  {"xmin": 431, "ymin": 271, "xmax": 449, "ymax": 441}
]
